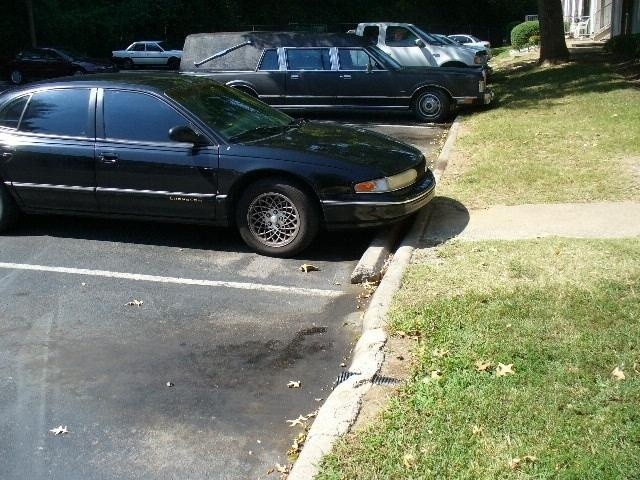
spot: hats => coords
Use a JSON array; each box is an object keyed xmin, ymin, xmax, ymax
[
  {"xmin": 0, "ymin": 69, "xmax": 435, "ymax": 256},
  {"xmin": 111, "ymin": 39, "xmax": 183, "ymax": 70},
  {"xmin": 0, "ymin": 45, "xmax": 121, "ymax": 85}
]
